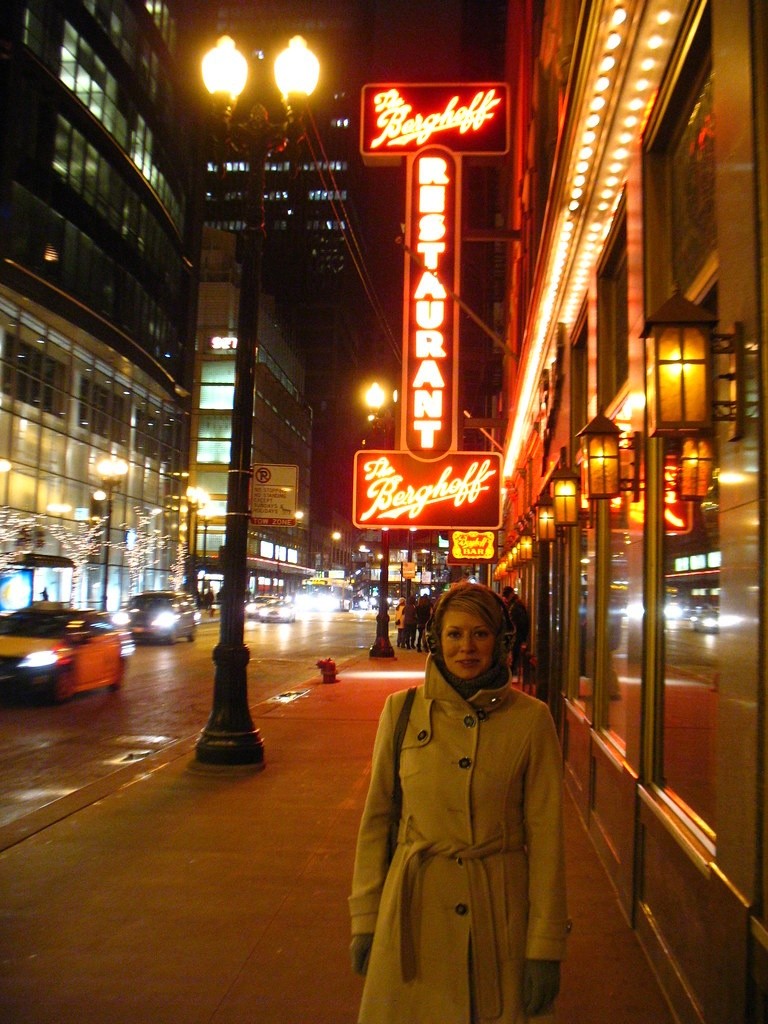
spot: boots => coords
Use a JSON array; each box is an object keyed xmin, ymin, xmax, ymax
[
  {"xmin": 411, "ymin": 639, "xmax": 416, "ymax": 649},
  {"xmin": 417, "ymin": 643, "xmax": 423, "ymax": 652},
  {"xmin": 424, "ymin": 643, "xmax": 429, "ymax": 652},
  {"xmin": 396, "ymin": 640, "xmax": 400, "ymax": 647},
  {"xmin": 405, "ymin": 639, "xmax": 411, "ymax": 649}
]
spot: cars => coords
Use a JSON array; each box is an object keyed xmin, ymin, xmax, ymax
[
  {"xmin": 352, "ymin": 596, "xmax": 368, "ymax": 609},
  {"xmin": 246, "ymin": 596, "xmax": 278, "ymax": 618},
  {"xmin": 664, "ymin": 602, "xmax": 719, "ymax": 632},
  {"xmin": 118, "ymin": 591, "xmax": 196, "ymax": 644},
  {"xmin": 371, "ymin": 594, "xmax": 397, "ymax": 610},
  {"xmin": 0, "ymin": 602, "xmax": 129, "ymax": 706},
  {"xmin": 259, "ymin": 600, "xmax": 295, "ymax": 622}
]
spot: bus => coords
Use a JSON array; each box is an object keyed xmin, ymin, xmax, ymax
[{"xmin": 304, "ymin": 578, "xmax": 353, "ymax": 611}]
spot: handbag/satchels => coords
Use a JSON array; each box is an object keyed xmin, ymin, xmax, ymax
[{"xmin": 395, "ymin": 619, "xmax": 400, "ymax": 625}]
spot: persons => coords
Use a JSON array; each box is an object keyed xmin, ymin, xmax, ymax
[
  {"xmin": 193, "ymin": 587, "xmax": 216, "ymax": 610},
  {"xmin": 348, "ymin": 583, "xmax": 569, "ymax": 1024},
  {"xmin": 394, "ymin": 587, "xmax": 530, "ymax": 684}
]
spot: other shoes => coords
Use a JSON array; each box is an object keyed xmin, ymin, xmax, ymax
[{"xmin": 511, "ymin": 676, "xmax": 519, "ymax": 683}]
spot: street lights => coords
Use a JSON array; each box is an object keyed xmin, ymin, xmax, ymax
[
  {"xmin": 194, "ymin": 34, "xmax": 321, "ymax": 761},
  {"xmin": 98, "ymin": 460, "xmax": 127, "ymax": 611},
  {"xmin": 329, "ymin": 533, "xmax": 340, "ymax": 569},
  {"xmin": 186, "ymin": 486, "xmax": 213, "ymax": 606},
  {"xmin": 363, "ymin": 381, "xmax": 396, "ymax": 656}
]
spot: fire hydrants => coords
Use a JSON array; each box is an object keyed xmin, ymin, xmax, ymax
[{"xmin": 316, "ymin": 658, "xmax": 339, "ymax": 683}]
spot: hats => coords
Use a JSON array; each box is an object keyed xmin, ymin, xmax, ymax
[
  {"xmin": 399, "ymin": 597, "xmax": 405, "ymax": 604},
  {"xmin": 502, "ymin": 586, "xmax": 514, "ymax": 597}
]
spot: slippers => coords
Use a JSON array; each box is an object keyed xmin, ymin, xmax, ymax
[{"xmin": 402, "ymin": 640, "xmax": 405, "ymax": 648}]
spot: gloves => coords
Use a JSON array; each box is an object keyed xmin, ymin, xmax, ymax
[
  {"xmin": 523, "ymin": 959, "xmax": 562, "ymax": 1017},
  {"xmin": 349, "ymin": 932, "xmax": 375, "ymax": 977}
]
